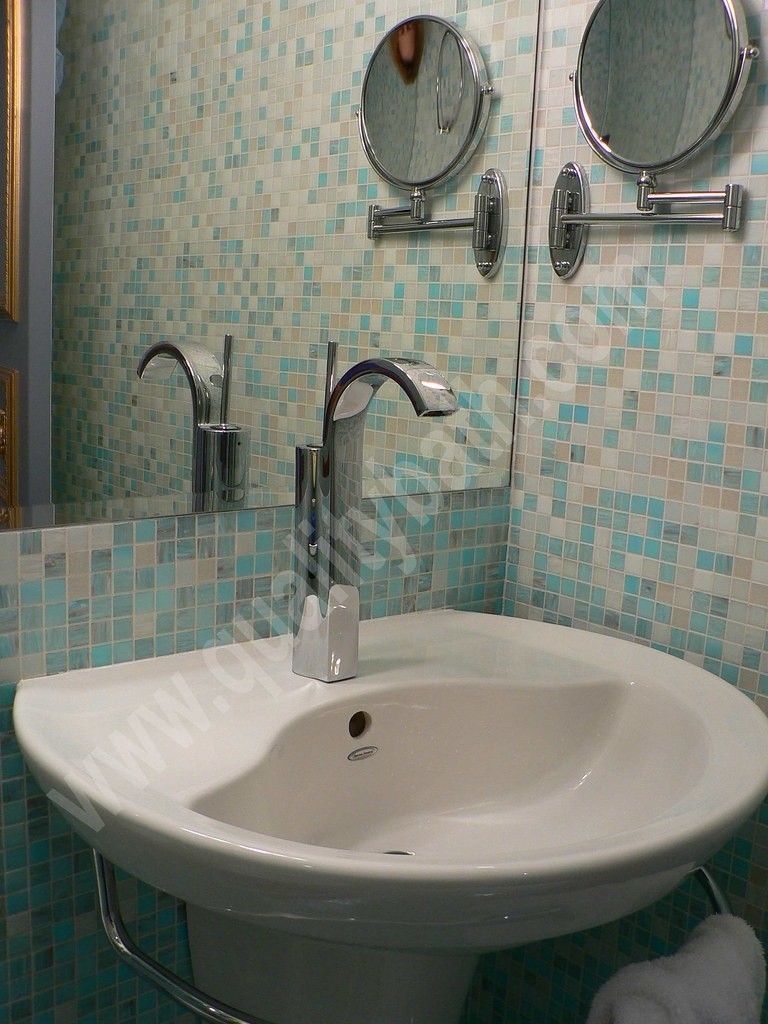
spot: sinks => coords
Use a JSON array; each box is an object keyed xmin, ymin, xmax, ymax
[{"xmin": 11, "ymin": 605, "xmax": 768, "ymax": 1024}]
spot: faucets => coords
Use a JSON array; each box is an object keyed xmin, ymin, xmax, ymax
[
  {"xmin": 291, "ymin": 340, "xmax": 461, "ymax": 684},
  {"xmin": 135, "ymin": 333, "xmax": 250, "ymax": 514}
]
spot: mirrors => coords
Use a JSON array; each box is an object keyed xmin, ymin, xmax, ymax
[
  {"xmin": 0, "ymin": 0, "xmax": 540, "ymax": 532},
  {"xmin": 548, "ymin": 0, "xmax": 759, "ymax": 279},
  {"xmin": 354, "ymin": 14, "xmax": 508, "ymax": 279}
]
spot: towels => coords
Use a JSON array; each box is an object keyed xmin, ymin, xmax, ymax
[{"xmin": 584, "ymin": 913, "xmax": 767, "ymax": 1024}]
